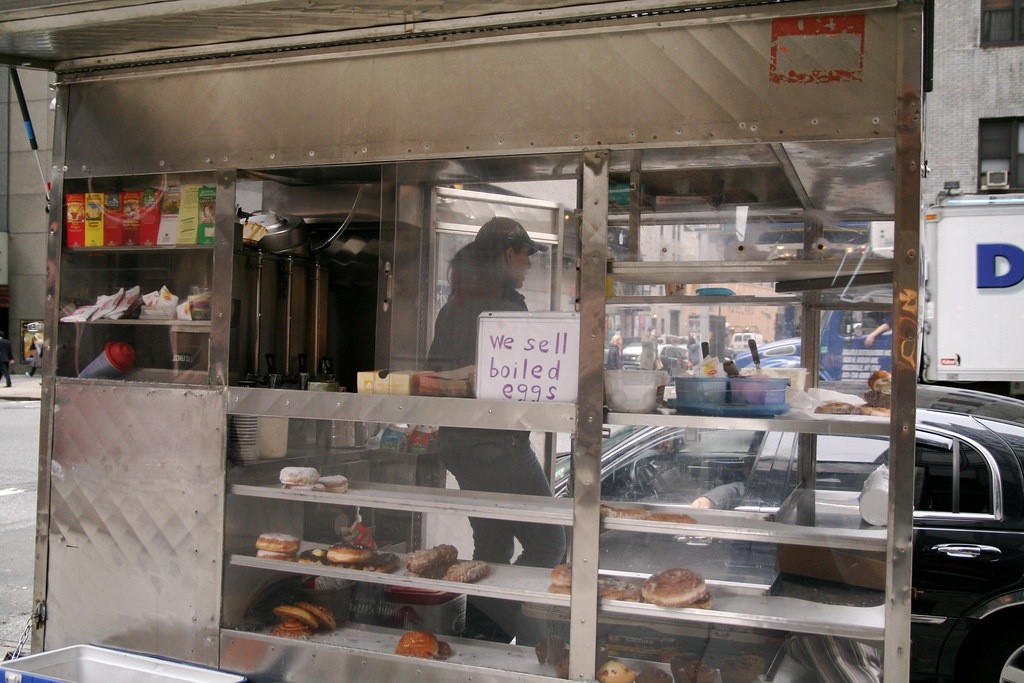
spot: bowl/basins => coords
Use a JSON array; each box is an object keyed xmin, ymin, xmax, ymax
[{"xmin": 604, "ymin": 367, "xmax": 807, "ymax": 413}]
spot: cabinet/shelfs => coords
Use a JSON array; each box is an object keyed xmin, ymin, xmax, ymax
[{"xmin": 30, "ymin": 1, "xmax": 924, "ymax": 683}]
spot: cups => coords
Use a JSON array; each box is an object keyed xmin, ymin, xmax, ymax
[{"xmin": 77, "ymin": 341, "xmax": 136, "ymax": 380}]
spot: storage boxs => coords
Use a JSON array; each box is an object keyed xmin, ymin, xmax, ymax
[
  {"xmin": 0, "ymin": 644, "xmax": 248, "ymax": 683},
  {"xmin": 65, "ymin": 185, "xmax": 217, "ymax": 247},
  {"xmin": 382, "ymin": 585, "xmax": 467, "ymax": 638},
  {"xmin": 357, "ymin": 365, "xmax": 476, "ymax": 399}
]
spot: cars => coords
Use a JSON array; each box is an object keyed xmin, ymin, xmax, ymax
[{"xmin": 551, "ymin": 334, "xmax": 1024, "ymax": 683}]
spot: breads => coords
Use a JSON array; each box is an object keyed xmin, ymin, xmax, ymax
[
  {"xmin": 535, "ymin": 505, "xmax": 769, "ymax": 683},
  {"xmin": 255, "ymin": 465, "xmax": 490, "ymax": 660},
  {"xmin": 813, "ymin": 369, "xmax": 894, "ymax": 416}
]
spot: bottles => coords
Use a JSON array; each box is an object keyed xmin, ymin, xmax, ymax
[{"xmin": 187, "ymin": 285, "xmax": 211, "ymax": 321}]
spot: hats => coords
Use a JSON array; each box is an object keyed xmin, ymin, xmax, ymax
[{"xmin": 474, "ymin": 217, "xmax": 549, "ymax": 255}]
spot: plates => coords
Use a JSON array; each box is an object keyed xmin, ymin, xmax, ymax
[{"xmin": 227, "ymin": 414, "xmax": 257, "ymax": 461}]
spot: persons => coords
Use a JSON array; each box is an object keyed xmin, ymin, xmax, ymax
[
  {"xmin": 0, "ymin": 322, "xmax": 45, "ymax": 388},
  {"xmin": 862, "ymin": 312, "xmax": 894, "ymax": 349},
  {"xmin": 605, "ymin": 321, "xmax": 698, "ymax": 379},
  {"xmin": 422, "ymin": 218, "xmax": 564, "ymax": 647},
  {"xmin": 691, "ymin": 430, "xmax": 766, "ymax": 510}
]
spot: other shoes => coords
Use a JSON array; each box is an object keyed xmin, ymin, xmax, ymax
[{"xmin": 26, "ymin": 372, "xmax": 32, "ymax": 379}]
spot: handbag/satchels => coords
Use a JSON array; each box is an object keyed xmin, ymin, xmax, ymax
[{"xmin": 654, "ymin": 358, "xmax": 664, "ymax": 371}]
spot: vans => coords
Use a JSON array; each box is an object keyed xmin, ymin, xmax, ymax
[{"xmin": 732, "ymin": 333, "xmax": 763, "ymax": 352}]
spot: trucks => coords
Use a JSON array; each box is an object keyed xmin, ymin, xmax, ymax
[{"xmin": 734, "ymin": 198, "xmax": 1024, "ymax": 385}]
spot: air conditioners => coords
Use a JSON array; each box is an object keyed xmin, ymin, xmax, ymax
[{"xmin": 987, "ymin": 170, "xmax": 1009, "ymax": 187}]
